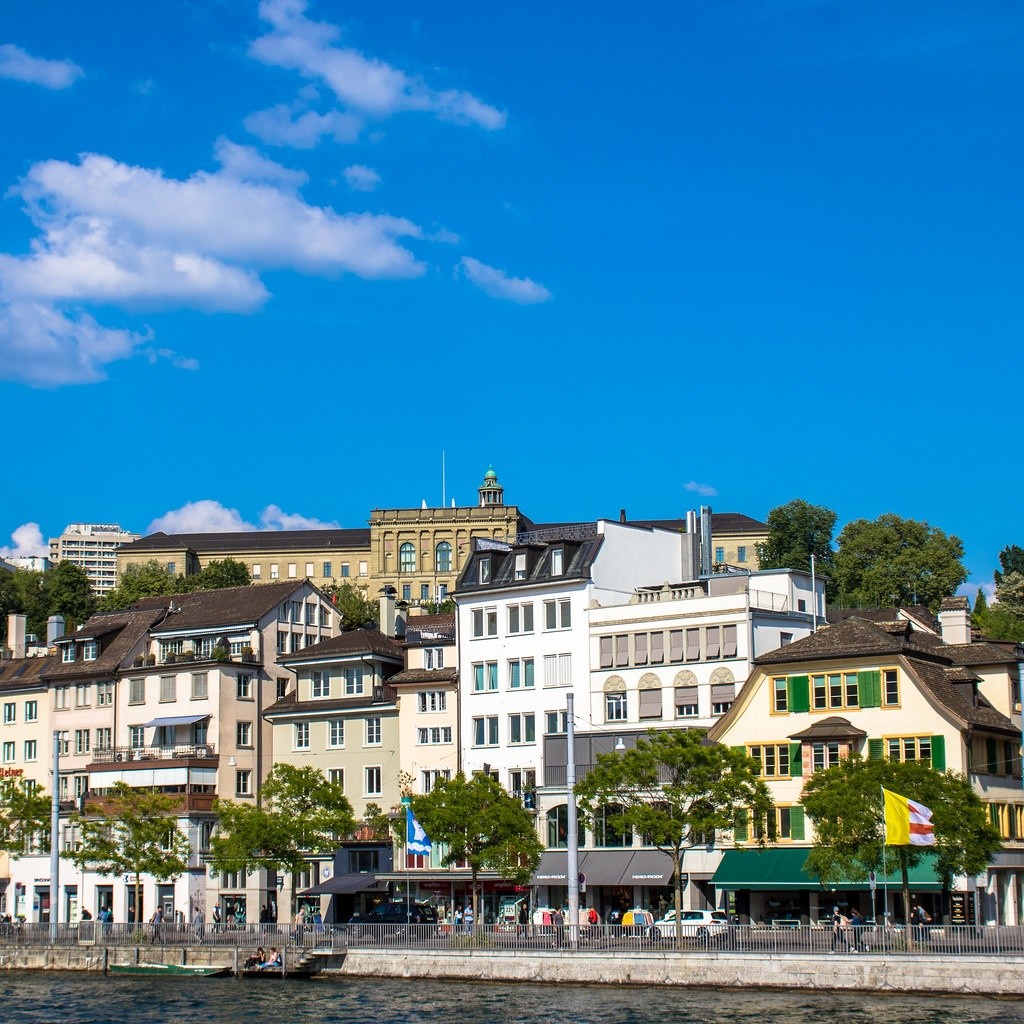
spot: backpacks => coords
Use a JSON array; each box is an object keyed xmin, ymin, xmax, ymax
[{"xmin": 836, "ymin": 915, "xmax": 850, "ymax": 929}]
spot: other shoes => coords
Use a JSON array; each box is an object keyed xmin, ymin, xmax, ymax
[
  {"xmin": 829, "ymin": 951, "xmax": 834, "ymax": 954},
  {"xmin": 866, "ymin": 946, "xmax": 869, "ymax": 952},
  {"xmin": 849, "ymin": 947, "xmax": 854, "ymax": 952}
]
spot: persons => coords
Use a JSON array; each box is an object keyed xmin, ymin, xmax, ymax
[
  {"xmin": 295, "ymin": 909, "xmax": 310, "ymax": 946},
  {"xmin": 98, "ymin": 906, "xmax": 113, "ymax": 938},
  {"xmin": 261, "ymin": 905, "xmax": 270, "ymax": 936},
  {"xmin": 80, "ymin": 906, "xmax": 87, "ymax": 919},
  {"xmin": 242, "ymin": 947, "xmax": 281, "ymax": 971},
  {"xmin": 586, "ymin": 905, "xmax": 599, "ymax": 941},
  {"xmin": 552, "ymin": 905, "xmax": 564, "ymax": 945},
  {"xmin": 828, "ymin": 907, "xmax": 854, "ymax": 954},
  {"xmin": 911, "ymin": 904, "xmax": 925, "ymax": 941},
  {"xmin": 621, "ymin": 891, "xmax": 632, "ymax": 911},
  {"xmin": 128, "ymin": 905, "xmax": 135, "ymax": 937},
  {"xmin": 843, "ymin": 910, "xmax": 870, "ymax": 953},
  {"xmin": 659, "ymin": 894, "xmax": 675, "ymax": 920},
  {"xmin": 211, "ymin": 902, "xmax": 221, "ymax": 935},
  {"xmin": 147, "ymin": 905, "xmax": 165, "ymax": 943},
  {"xmin": 774, "ymin": 913, "xmax": 778, "ymax": 919},
  {"xmin": 517, "ymin": 903, "xmax": 529, "ymax": 939},
  {"xmin": 193, "ymin": 907, "xmax": 203, "ymax": 946},
  {"xmin": 454, "ymin": 904, "xmax": 474, "ymax": 936}
]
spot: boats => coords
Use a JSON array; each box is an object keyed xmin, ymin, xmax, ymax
[{"xmin": 109, "ymin": 960, "xmax": 232, "ymax": 977}]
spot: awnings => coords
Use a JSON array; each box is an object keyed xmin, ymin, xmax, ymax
[
  {"xmin": 297, "ymin": 875, "xmax": 380, "ymax": 894},
  {"xmin": 708, "ymin": 850, "xmax": 954, "ymax": 890},
  {"xmin": 141, "ymin": 714, "xmax": 209, "ymax": 728},
  {"xmin": 528, "ymin": 850, "xmax": 685, "ymax": 886}
]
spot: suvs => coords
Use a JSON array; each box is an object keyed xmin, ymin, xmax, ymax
[
  {"xmin": 645, "ymin": 910, "xmax": 729, "ymax": 942},
  {"xmin": 348, "ymin": 902, "xmax": 438, "ymax": 939}
]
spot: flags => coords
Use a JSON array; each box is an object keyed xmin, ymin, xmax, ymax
[
  {"xmin": 407, "ymin": 809, "xmax": 432, "ymax": 855},
  {"xmin": 884, "ymin": 788, "xmax": 936, "ymax": 846}
]
